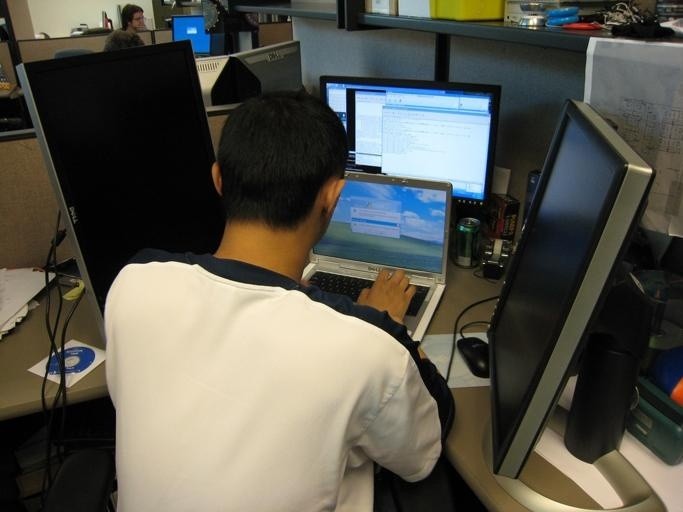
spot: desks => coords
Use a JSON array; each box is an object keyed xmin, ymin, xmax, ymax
[{"xmin": 0, "ymin": 257, "xmax": 683, "ymax": 512}]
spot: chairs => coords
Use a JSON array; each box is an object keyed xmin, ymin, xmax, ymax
[{"xmin": 53, "ymin": 48, "xmax": 93, "ymax": 58}]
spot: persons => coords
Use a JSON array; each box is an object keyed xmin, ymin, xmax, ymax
[
  {"xmin": 120, "ymin": 4, "xmax": 145, "ymax": 34},
  {"xmin": 102, "ymin": 88, "xmax": 455, "ymax": 512},
  {"xmin": 102, "ymin": 29, "xmax": 145, "ymax": 52}
]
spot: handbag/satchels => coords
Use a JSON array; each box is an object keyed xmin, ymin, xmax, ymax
[{"xmin": 565, "ymin": 284, "xmax": 654, "ymax": 462}]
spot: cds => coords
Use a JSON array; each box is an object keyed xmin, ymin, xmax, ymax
[{"xmin": 45, "ymin": 345, "xmax": 96, "ymax": 376}]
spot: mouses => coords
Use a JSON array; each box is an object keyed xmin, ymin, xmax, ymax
[{"xmin": 456, "ymin": 337, "xmax": 490, "ymax": 379}]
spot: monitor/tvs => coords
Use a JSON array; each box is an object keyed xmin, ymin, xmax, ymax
[
  {"xmin": 210, "ymin": 39, "xmax": 303, "ymax": 106},
  {"xmin": 171, "ymin": 14, "xmax": 211, "ymax": 56},
  {"xmin": 318, "ymin": 74, "xmax": 502, "ymax": 210},
  {"xmin": 12, "ymin": 39, "xmax": 228, "ymax": 344},
  {"xmin": 194, "ymin": 54, "xmax": 243, "ymax": 115},
  {"xmin": 102, "ymin": 10, "xmax": 113, "ymax": 30},
  {"xmin": 485, "ymin": 98, "xmax": 665, "ymax": 512}
]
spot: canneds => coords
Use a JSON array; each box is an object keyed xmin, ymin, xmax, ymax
[{"xmin": 453, "ymin": 218, "xmax": 481, "ymax": 269}]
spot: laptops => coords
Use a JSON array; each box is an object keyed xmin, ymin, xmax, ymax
[{"xmin": 301, "ymin": 170, "xmax": 453, "ymax": 345}]
